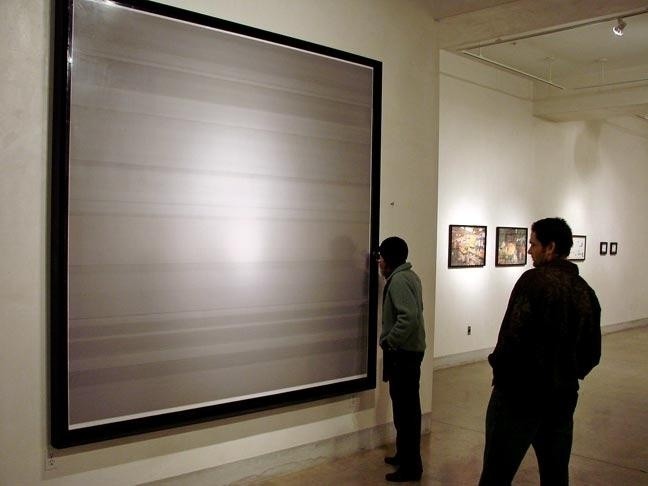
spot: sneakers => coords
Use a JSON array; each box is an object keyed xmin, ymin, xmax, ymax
[{"xmin": 384, "ymin": 455, "xmax": 421, "ymax": 482}]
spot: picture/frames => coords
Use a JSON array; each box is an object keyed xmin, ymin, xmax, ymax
[
  {"xmin": 495, "ymin": 226, "xmax": 528, "ymax": 267},
  {"xmin": 566, "ymin": 235, "xmax": 587, "ymax": 261},
  {"xmin": 610, "ymin": 242, "xmax": 617, "ymax": 254},
  {"xmin": 600, "ymin": 242, "xmax": 607, "ymax": 255},
  {"xmin": 46, "ymin": 0, "xmax": 383, "ymax": 452},
  {"xmin": 447, "ymin": 224, "xmax": 487, "ymax": 268}
]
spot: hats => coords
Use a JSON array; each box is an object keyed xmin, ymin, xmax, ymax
[{"xmin": 377, "ymin": 237, "xmax": 408, "ymax": 257}]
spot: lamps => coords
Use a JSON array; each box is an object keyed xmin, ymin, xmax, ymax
[{"xmin": 612, "ymin": 16, "xmax": 628, "ymax": 37}]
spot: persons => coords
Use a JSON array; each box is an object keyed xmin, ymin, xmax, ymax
[
  {"xmin": 379, "ymin": 239, "xmax": 425, "ymax": 481},
  {"xmin": 477, "ymin": 218, "xmax": 601, "ymax": 486}
]
